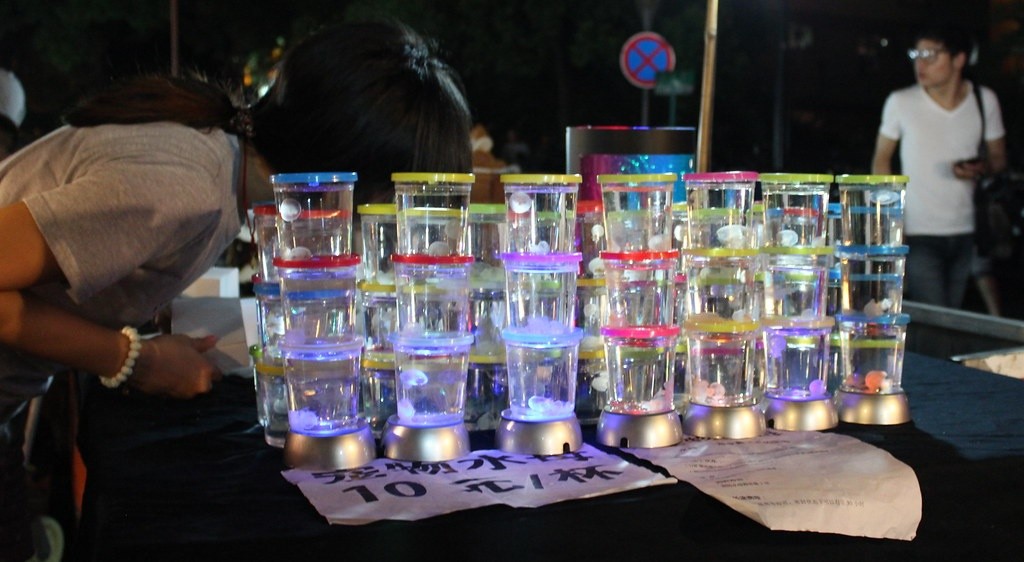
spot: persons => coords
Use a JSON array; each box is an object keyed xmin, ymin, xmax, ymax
[
  {"xmin": 871, "ymin": 17, "xmax": 1007, "ymax": 355},
  {"xmin": 0, "ymin": 17, "xmax": 474, "ymax": 562}
]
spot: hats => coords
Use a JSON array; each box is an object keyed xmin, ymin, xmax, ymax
[{"xmin": 0, "ymin": 68, "xmax": 28, "ymax": 128}]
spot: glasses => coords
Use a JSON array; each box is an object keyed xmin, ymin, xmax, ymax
[{"xmin": 907, "ymin": 44, "xmax": 946, "ymax": 64}]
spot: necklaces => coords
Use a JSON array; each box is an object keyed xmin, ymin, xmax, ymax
[{"xmin": 238, "ymin": 142, "xmax": 260, "ymax": 255}]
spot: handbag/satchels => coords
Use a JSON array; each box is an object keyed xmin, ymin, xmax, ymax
[
  {"xmin": 23, "ymin": 367, "xmax": 86, "ymax": 524},
  {"xmin": 974, "ymin": 173, "xmax": 1024, "ymax": 241}
]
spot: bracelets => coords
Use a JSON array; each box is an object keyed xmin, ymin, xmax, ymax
[{"xmin": 96, "ymin": 324, "xmax": 142, "ymax": 391}]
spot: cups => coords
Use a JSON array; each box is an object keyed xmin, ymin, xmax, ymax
[{"xmin": 250, "ymin": 174, "xmax": 912, "ymax": 451}]
